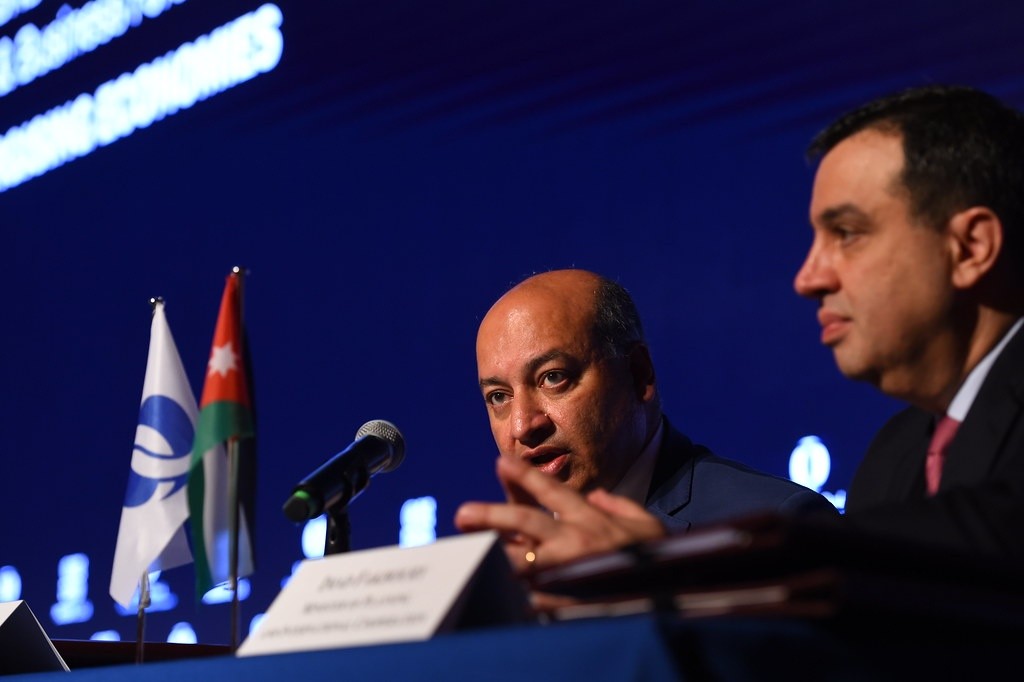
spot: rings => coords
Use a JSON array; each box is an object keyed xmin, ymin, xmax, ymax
[{"xmin": 527, "ymin": 542, "xmax": 536, "ymax": 581}]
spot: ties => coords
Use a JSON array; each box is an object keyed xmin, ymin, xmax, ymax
[{"xmin": 924, "ymin": 414, "xmax": 961, "ymax": 496}]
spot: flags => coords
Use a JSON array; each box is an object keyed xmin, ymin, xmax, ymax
[
  {"xmin": 186, "ymin": 271, "xmax": 261, "ymax": 599},
  {"xmin": 109, "ymin": 303, "xmax": 199, "ymax": 611}
]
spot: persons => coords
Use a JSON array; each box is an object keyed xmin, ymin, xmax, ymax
[
  {"xmin": 475, "ymin": 269, "xmax": 838, "ymax": 540},
  {"xmin": 449, "ymin": 92, "xmax": 1024, "ymax": 615}
]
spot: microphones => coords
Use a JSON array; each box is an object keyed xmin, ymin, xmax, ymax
[{"xmin": 281, "ymin": 419, "xmax": 405, "ymax": 524}]
